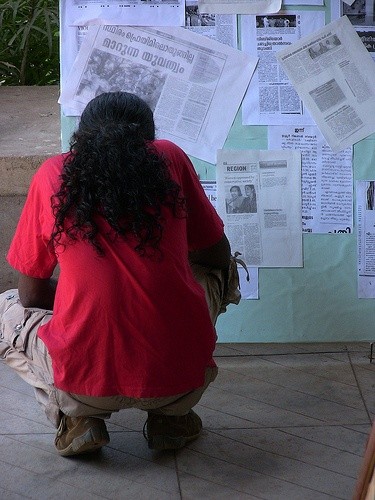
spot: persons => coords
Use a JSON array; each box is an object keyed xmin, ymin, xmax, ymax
[
  {"xmin": 258, "ymin": 16, "xmax": 291, "ymax": 27},
  {"xmin": 0, "ymin": 92, "xmax": 231, "ymax": 456},
  {"xmin": 309, "ymin": 36, "xmax": 340, "ymax": 58},
  {"xmin": 227, "ymin": 185, "xmax": 257, "ymax": 214},
  {"xmin": 360, "ymin": 34, "xmax": 375, "ymax": 48}
]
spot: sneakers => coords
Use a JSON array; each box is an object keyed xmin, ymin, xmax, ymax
[
  {"xmin": 143, "ymin": 409, "xmax": 202, "ymax": 449},
  {"xmin": 53, "ymin": 414, "xmax": 110, "ymax": 456}
]
